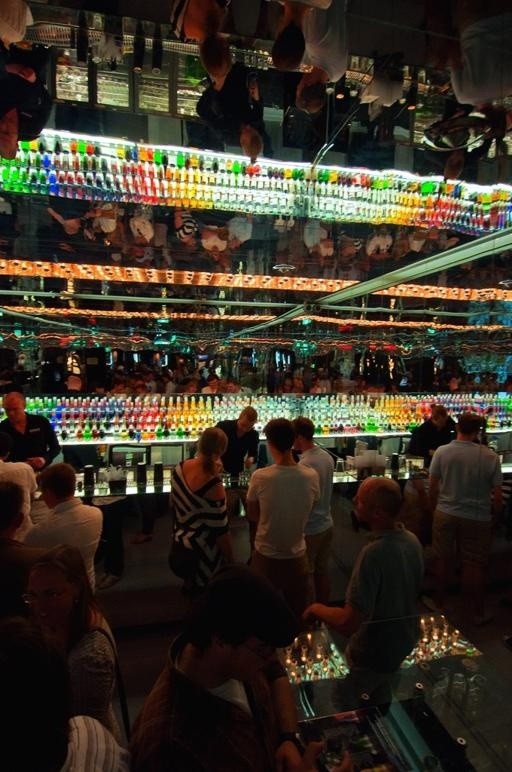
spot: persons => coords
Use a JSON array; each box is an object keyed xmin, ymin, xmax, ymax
[
  {"xmin": 0, "ymin": 344, "xmax": 512, "ymax": 772},
  {"xmin": 0, "ymin": 2, "xmax": 511, "ymax": 334}
]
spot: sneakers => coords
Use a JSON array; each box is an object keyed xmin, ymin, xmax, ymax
[{"xmin": 422, "ymin": 595, "xmax": 439, "ymax": 611}]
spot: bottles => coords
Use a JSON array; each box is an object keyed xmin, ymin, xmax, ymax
[
  {"xmin": 413, "ymin": 618, "xmax": 480, "ymax": 665},
  {"xmin": 1, "ymin": 134, "xmax": 512, "ymax": 241},
  {"xmin": 228, "ymin": 44, "xmax": 269, "ymax": 72},
  {"xmin": 0, "ymin": 389, "xmax": 512, "ymax": 441},
  {"xmin": 283, "ymin": 640, "xmax": 344, "ymax": 685}
]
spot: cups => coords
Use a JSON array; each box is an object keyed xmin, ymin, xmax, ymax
[
  {"xmin": 335, "ymin": 460, "xmax": 345, "ymax": 477},
  {"xmin": 83, "ymin": 465, "xmax": 95, "ymax": 489},
  {"xmin": 97, "ymin": 468, "xmax": 108, "ymax": 488},
  {"xmin": 153, "ymin": 462, "xmax": 165, "ymax": 483},
  {"xmin": 222, "ymin": 471, "xmax": 251, "ymax": 485},
  {"xmin": 136, "ymin": 462, "xmax": 148, "ymax": 483},
  {"xmin": 355, "ymin": 448, "xmax": 386, "ymax": 478},
  {"xmin": 390, "ymin": 452, "xmax": 399, "ymax": 471}
]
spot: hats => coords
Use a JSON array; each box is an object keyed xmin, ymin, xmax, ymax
[{"xmin": 205, "ymin": 565, "xmax": 300, "ymax": 648}]
